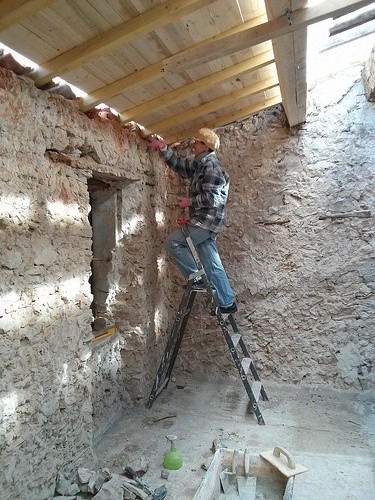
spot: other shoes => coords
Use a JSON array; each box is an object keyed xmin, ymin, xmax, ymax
[
  {"xmin": 182, "ymin": 277, "xmax": 205, "ymax": 290},
  {"xmin": 211, "ymin": 302, "xmax": 237, "ymax": 313}
]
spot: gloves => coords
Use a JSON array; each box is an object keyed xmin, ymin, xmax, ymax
[
  {"xmin": 146, "ymin": 136, "xmax": 166, "ymax": 150},
  {"xmin": 176, "ymin": 197, "xmax": 192, "ymax": 208}
]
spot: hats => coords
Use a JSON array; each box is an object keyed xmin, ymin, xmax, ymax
[{"xmin": 192, "ymin": 127, "xmax": 220, "ymax": 151}]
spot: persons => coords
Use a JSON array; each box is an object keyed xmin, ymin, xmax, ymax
[{"xmin": 145, "ymin": 128, "xmax": 239, "ymax": 316}]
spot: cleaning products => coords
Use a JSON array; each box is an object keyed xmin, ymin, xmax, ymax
[{"xmin": 163, "ymin": 434, "xmax": 183, "ymax": 470}]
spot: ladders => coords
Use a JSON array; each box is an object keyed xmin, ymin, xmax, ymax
[{"xmin": 146, "ymin": 225, "xmax": 269, "ymax": 426}]
